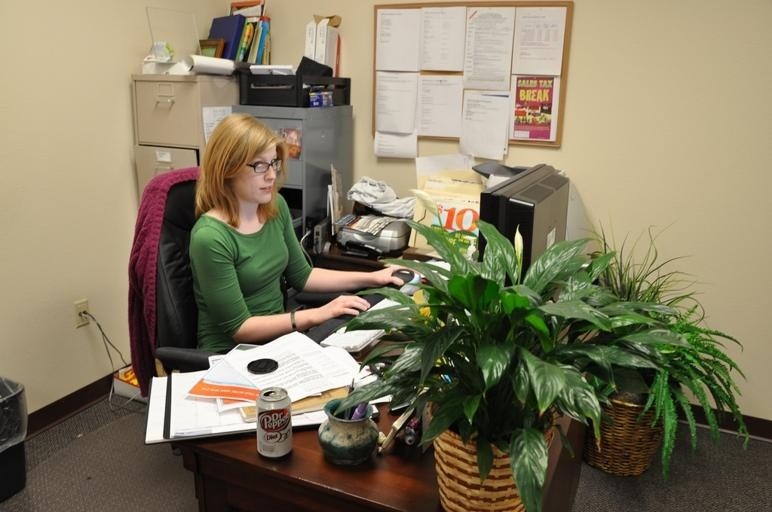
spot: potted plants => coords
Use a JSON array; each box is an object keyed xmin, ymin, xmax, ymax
[
  {"xmin": 334, "ymin": 189, "xmax": 693, "ymax": 512},
  {"xmin": 559, "ymin": 219, "xmax": 749, "ymax": 480}
]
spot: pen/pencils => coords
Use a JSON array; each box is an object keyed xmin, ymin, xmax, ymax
[{"xmin": 344, "ymin": 378, "xmax": 355, "ymax": 419}]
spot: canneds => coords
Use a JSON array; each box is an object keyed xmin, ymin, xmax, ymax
[{"xmin": 254, "ymin": 385, "xmax": 293, "ymax": 458}]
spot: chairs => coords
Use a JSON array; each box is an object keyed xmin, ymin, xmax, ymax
[{"xmin": 127, "ymin": 167, "xmax": 353, "ymax": 456}]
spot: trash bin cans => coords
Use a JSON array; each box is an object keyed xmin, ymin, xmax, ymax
[{"xmin": 0, "ymin": 376, "xmax": 27, "ymax": 503}]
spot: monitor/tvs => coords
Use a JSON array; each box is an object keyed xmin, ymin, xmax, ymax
[{"xmin": 476, "ymin": 163, "xmax": 572, "ymax": 287}]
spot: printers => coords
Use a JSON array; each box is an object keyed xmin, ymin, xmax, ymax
[{"xmin": 335, "ymin": 216, "xmax": 410, "ymax": 260}]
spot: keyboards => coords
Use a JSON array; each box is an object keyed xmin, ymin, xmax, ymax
[{"xmin": 317, "ymin": 294, "xmax": 419, "ymax": 353}]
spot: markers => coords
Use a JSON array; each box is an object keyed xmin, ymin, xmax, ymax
[{"xmin": 351, "ymin": 399, "xmax": 369, "ymax": 419}]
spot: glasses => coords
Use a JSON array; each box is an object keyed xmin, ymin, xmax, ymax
[{"xmin": 245, "ymin": 158, "xmax": 281, "ymax": 173}]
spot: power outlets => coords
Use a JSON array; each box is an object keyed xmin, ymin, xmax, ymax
[{"xmin": 74, "ymin": 299, "xmax": 90, "ymax": 328}]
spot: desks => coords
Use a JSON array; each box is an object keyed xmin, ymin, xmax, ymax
[{"xmin": 183, "ymin": 341, "xmax": 582, "ymax": 512}]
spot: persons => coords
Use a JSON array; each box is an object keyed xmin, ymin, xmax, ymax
[{"xmin": 188, "ymin": 112, "xmax": 415, "ymax": 353}]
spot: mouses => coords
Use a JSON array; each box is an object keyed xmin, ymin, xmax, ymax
[{"xmin": 392, "ymin": 269, "xmax": 416, "ymax": 281}]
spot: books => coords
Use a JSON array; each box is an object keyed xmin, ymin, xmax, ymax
[{"xmin": 207, "ymin": 14, "xmax": 271, "ymax": 65}]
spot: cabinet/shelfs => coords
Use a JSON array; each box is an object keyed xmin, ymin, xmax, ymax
[
  {"xmin": 232, "ymin": 104, "xmax": 352, "ymax": 237},
  {"xmin": 133, "ymin": 74, "xmax": 239, "ymax": 205}
]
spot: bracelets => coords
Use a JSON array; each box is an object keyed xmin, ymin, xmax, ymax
[{"xmin": 291, "ymin": 312, "xmax": 296, "ymax": 330}]
